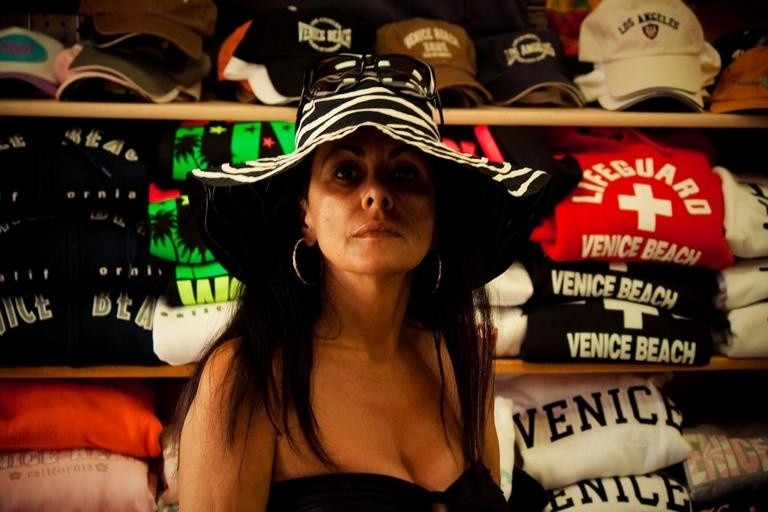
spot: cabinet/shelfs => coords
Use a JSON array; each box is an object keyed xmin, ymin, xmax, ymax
[{"xmin": 0, "ymin": 99, "xmax": 768, "ymax": 379}]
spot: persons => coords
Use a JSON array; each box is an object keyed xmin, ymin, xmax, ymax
[{"xmin": 176, "ymin": 50, "xmax": 552, "ymax": 512}]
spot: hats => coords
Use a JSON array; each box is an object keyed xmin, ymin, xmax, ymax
[
  {"xmin": 186, "ymin": 52, "xmax": 581, "ymax": 289},
  {"xmin": 0, "ymin": 1, "xmax": 768, "ymax": 114}
]
